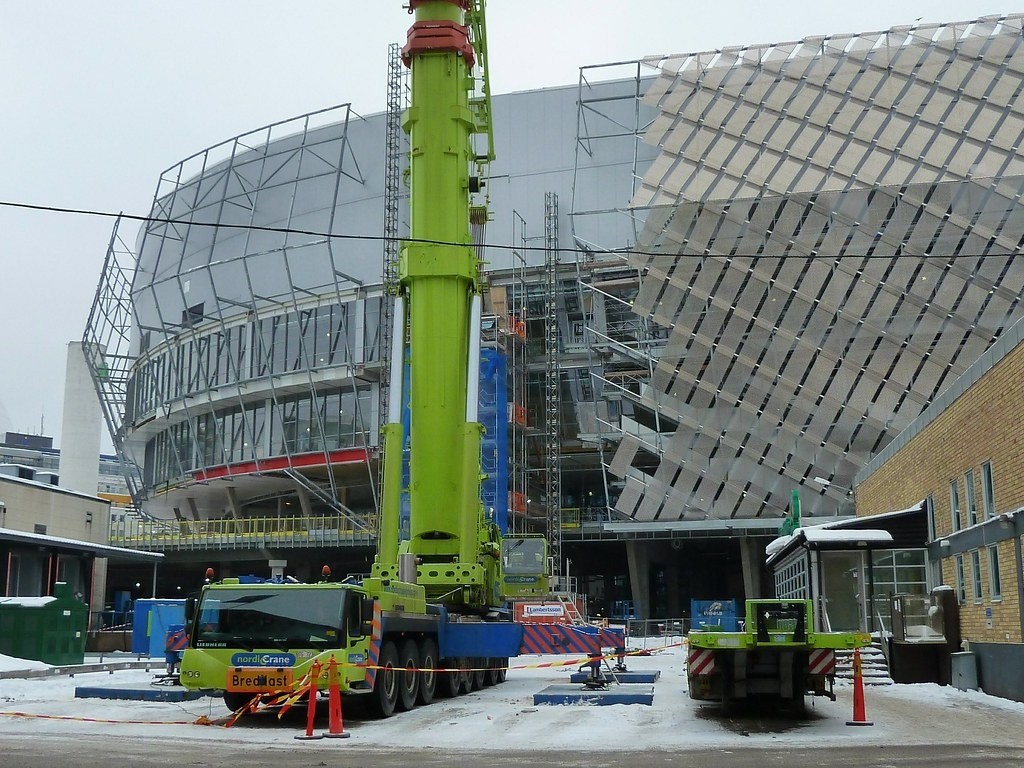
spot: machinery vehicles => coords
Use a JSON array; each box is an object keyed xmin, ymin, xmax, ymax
[
  {"xmin": 152, "ymin": 0, "xmax": 628, "ymax": 727},
  {"xmin": 686, "ymin": 598, "xmax": 873, "ymax": 718}
]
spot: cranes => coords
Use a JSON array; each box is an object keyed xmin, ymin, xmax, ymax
[{"xmin": 486, "ymin": 190, "xmax": 585, "ymax": 625}]
